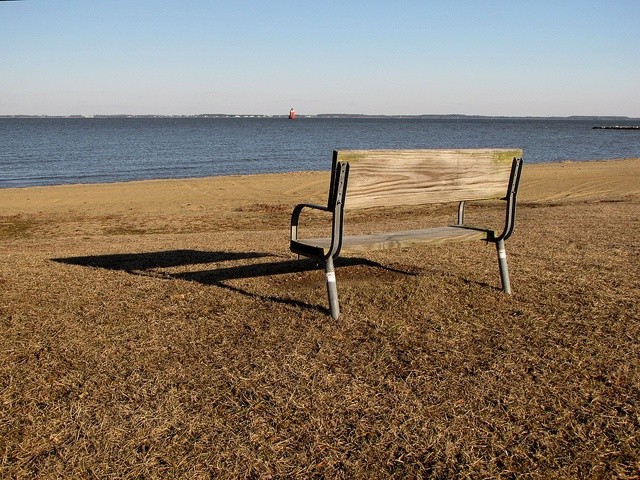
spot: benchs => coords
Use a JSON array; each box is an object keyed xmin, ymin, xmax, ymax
[{"xmin": 288, "ymin": 148, "xmax": 526, "ymax": 321}]
